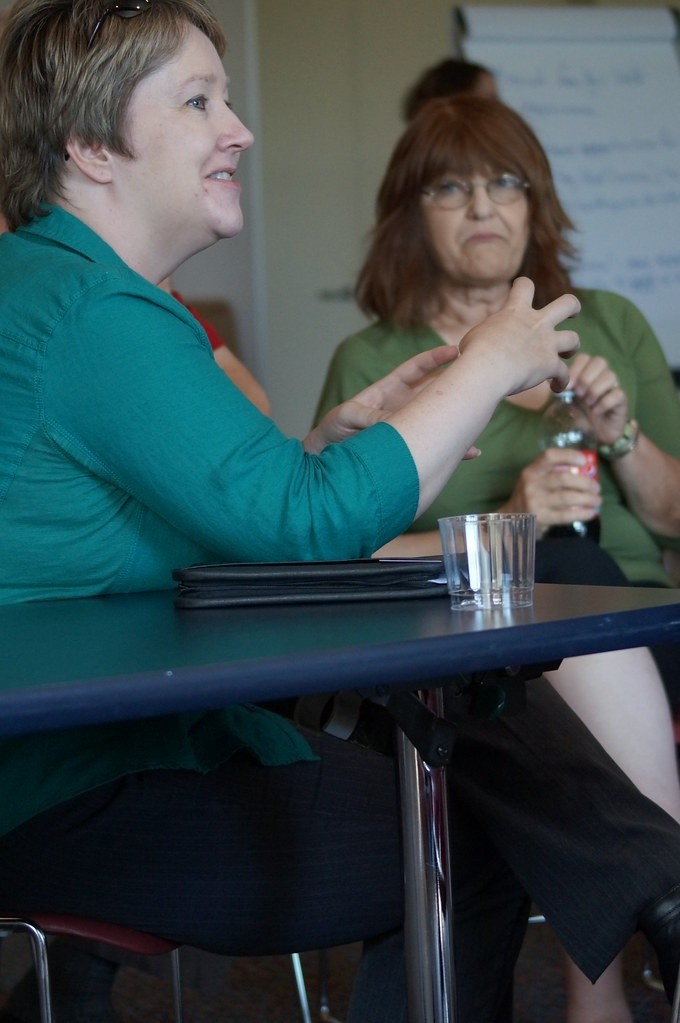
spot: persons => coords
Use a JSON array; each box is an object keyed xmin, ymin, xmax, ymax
[
  {"xmin": 311, "ymin": 95, "xmax": 680, "ymax": 1023},
  {"xmin": 161, "ymin": 281, "xmax": 273, "ymax": 428},
  {"xmin": 0, "ymin": 0, "xmax": 680, "ymax": 1023},
  {"xmin": 399, "ymin": 60, "xmax": 498, "ymax": 123}
]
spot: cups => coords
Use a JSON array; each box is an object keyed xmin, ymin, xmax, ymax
[
  {"xmin": 489, "ymin": 513, "xmax": 535, "ymax": 609},
  {"xmin": 437, "ymin": 512, "xmax": 492, "ymax": 613}
]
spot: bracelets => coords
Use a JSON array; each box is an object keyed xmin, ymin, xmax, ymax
[{"xmin": 597, "ymin": 419, "xmax": 640, "ymax": 460}]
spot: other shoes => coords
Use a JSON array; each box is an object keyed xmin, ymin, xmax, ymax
[{"xmin": 640, "ymin": 885, "xmax": 680, "ymax": 1004}]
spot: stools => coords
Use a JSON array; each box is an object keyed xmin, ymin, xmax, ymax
[{"xmin": 0, "ymin": 909, "xmax": 313, "ymax": 1022}]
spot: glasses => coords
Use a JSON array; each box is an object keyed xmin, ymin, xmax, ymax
[
  {"xmin": 87, "ymin": 0, "xmax": 151, "ymax": 52},
  {"xmin": 419, "ymin": 173, "xmax": 531, "ymax": 210}
]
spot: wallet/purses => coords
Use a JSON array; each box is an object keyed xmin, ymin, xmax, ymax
[{"xmin": 173, "ymin": 552, "xmax": 466, "ymax": 607}]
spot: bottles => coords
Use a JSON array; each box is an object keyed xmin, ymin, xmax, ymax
[{"xmin": 536, "ymin": 390, "xmax": 604, "ymax": 577}]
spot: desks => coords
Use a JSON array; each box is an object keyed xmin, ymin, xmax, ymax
[{"xmin": 1, "ymin": 585, "xmax": 680, "ymax": 1022}]
